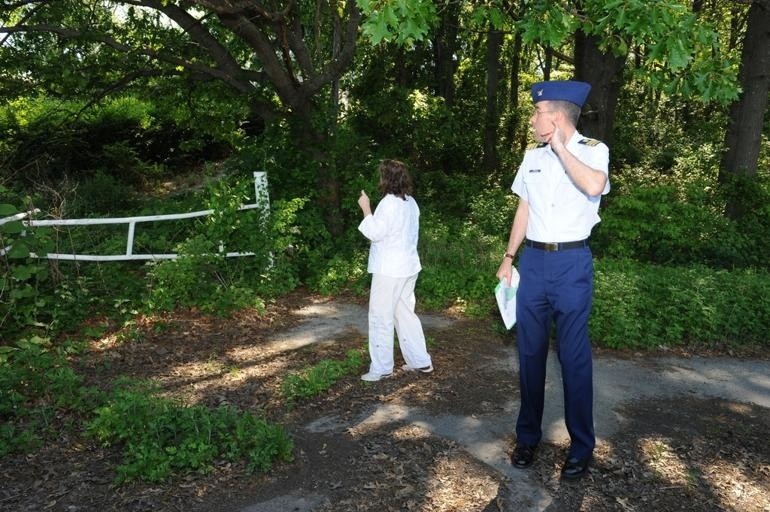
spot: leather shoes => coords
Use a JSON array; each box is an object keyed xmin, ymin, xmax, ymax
[
  {"xmin": 560, "ymin": 450, "xmax": 593, "ymax": 477},
  {"xmin": 512, "ymin": 440, "xmax": 538, "ymax": 469}
]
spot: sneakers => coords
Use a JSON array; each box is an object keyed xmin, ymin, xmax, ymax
[
  {"xmin": 360, "ymin": 372, "xmax": 394, "ymax": 382},
  {"xmin": 401, "ymin": 364, "xmax": 434, "ymax": 373}
]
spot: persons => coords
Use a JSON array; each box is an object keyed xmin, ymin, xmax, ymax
[
  {"xmin": 495, "ymin": 81, "xmax": 611, "ymax": 479},
  {"xmin": 356, "ymin": 159, "xmax": 434, "ymax": 381}
]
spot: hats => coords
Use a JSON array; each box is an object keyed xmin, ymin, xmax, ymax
[{"xmin": 531, "ymin": 80, "xmax": 592, "ymax": 108}]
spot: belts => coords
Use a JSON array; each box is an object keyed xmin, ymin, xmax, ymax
[{"xmin": 524, "ymin": 239, "xmax": 588, "ymax": 252}]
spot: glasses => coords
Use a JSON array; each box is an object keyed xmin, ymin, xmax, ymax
[{"xmin": 532, "ymin": 108, "xmax": 556, "ymax": 119}]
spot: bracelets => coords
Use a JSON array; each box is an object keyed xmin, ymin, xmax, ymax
[{"xmin": 503, "ymin": 253, "xmax": 514, "ymax": 260}]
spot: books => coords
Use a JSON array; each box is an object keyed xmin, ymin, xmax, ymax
[{"xmin": 494, "ymin": 266, "xmax": 520, "ymax": 332}]
